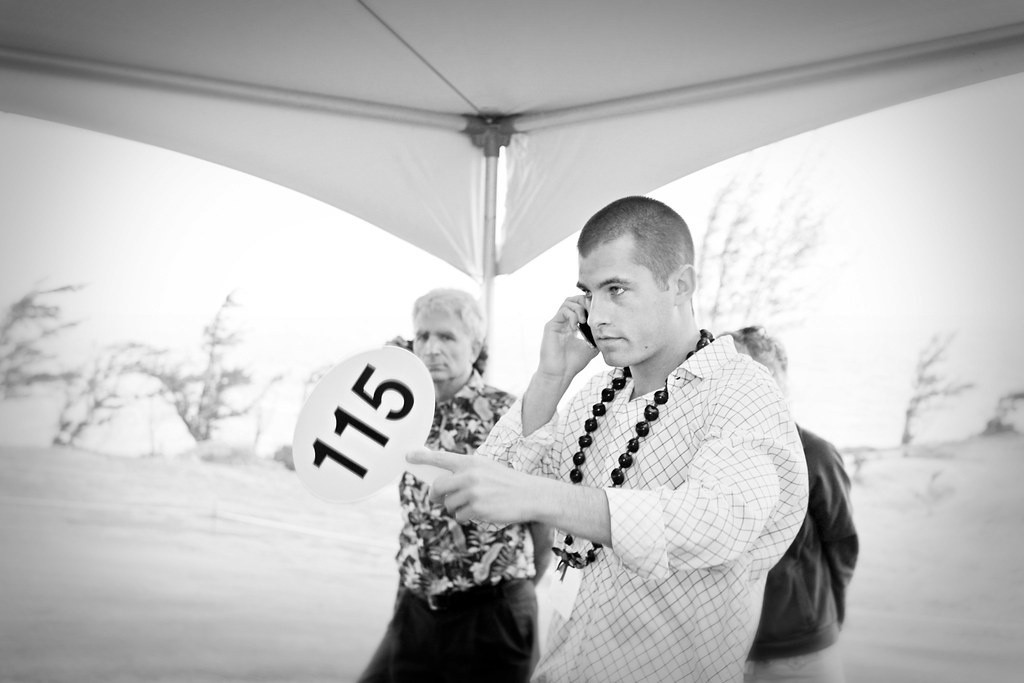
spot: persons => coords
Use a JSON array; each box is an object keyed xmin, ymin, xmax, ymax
[
  {"xmin": 407, "ymin": 196, "xmax": 809, "ymax": 682},
  {"xmin": 716, "ymin": 327, "xmax": 859, "ymax": 682},
  {"xmin": 354, "ymin": 290, "xmax": 555, "ymax": 683}
]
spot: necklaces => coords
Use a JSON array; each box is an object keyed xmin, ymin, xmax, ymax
[{"xmin": 551, "ymin": 329, "xmax": 713, "ymax": 581}]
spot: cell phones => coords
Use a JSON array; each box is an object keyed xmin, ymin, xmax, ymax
[{"xmin": 577, "ymin": 309, "xmax": 597, "ymax": 348}]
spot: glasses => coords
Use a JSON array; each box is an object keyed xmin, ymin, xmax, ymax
[{"xmin": 736, "ymin": 325, "xmax": 767, "ymax": 339}]
